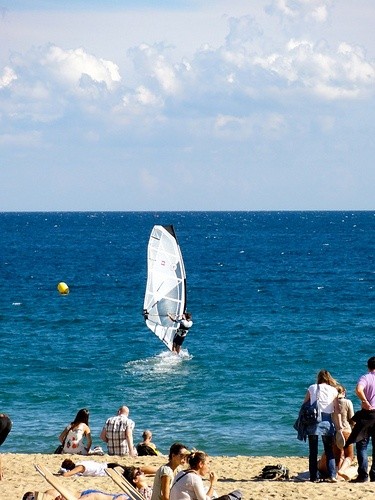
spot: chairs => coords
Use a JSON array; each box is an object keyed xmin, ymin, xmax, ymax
[
  {"xmin": 34, "ymin": 463, "xmax": 80, "ymax": 500},
  {"xmin": 104, "ymin": 468, "xmax": 146, "ymax": 500}
]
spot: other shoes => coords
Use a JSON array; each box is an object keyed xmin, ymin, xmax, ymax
[{"xmin": 350, "ymin": 475, "xmax": 368, "ymax": 483}]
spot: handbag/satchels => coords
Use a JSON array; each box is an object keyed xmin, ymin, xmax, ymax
[
  {"xmin": 54, "ymin": 445, "xmax": 64, "ymax": 454},
  {"xmin": 300, "ymin": 399, "xmax": 322, "ymax": 425}
]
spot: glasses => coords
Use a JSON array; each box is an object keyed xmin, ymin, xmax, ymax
[
  {"xmin": 178, "ymin": 454, "xmax": 189, "ymax": 457},
  {"xmin": 135, "ymin": 469, "xmax": 141, "ymax": 478}
]
